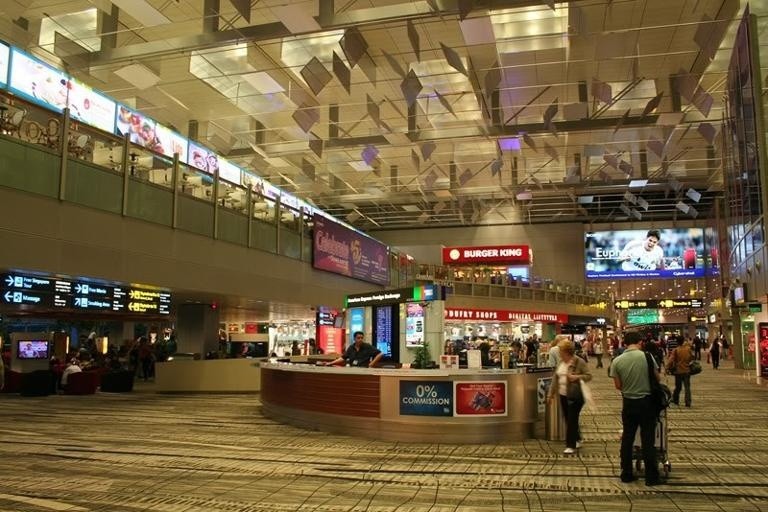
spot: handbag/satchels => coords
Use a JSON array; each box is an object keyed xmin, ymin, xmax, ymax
[
  {"xmin": 691, "ymin": 362, "xmax": 702, "ymax": 375},
  {"xmin": 568, "ymin": 380, "xmax": 585, "ymax": 404},
  {"xmin": 652, "ymin": 384, "xmax": 672, "ymax": 410}
]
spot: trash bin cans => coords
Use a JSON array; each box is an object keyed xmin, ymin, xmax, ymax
[{"xmin": 545, "ymin": 384, "xmax": 567, "ymax": 441}]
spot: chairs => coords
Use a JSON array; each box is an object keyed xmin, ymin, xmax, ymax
[
  {"xmin": 0, "ymin": 101, "xmax": 294, "ymax": 228},
  {"xmin": 2, "ymin": 350, "xmax": 136, "ymax": 396}
]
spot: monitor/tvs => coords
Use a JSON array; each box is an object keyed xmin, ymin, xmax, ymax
[
  {"xmin": 333, "ymin": 314, "xmax": 344, "ymax": 328},
  {"xmin": 17, "ymin": 340, "xmax": 49, "ymax": 360},
  {"xmin": 507, "ymin": 265, "xmax": 529, "ymax": 282}
]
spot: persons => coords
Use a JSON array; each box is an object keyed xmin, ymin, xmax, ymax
[
  {"xmin": 235, "ymin": 342, "xmax": 249, "ymax": 358},
  {"xmin": 292, "ymin": 340, "xmax": 300, "ymax": 356},
  {"xmin": 546, "ymin": 339, "xmax": 593, "ymax": 453},
  {"xmin": 609, "ymin": 331, "xmax": 667, "ymax": 486},
  {"xmin": 639, "ymin": 332, "xmax": 679, "ymax": 374},
  {"xmin": 617, "ymin": 229, "xmax": 665, "ymax": 271},
  {"xmin": 61, "ymin": 338, "xmax": 151, "ymax": 391},
  {"xmin": 443, "ymin": 334, "xmax": 548, "ymax": 369},
  {"xmin": 21, "ymin": 344, "xmax": 39, "ymax": 358},
  {"xmin": 669, "ymin": 335, "xmax": 693, "ymax": 407},
  {"xmin": 574, "ymin": 332, "xmax": 626, "ymax": 369},
  {"xmin": 683, "ymin": 331, "xmax": 728, "ymax": 370},
  {"xmin": 310, "ymin": 338, "xmax": 323, "ymax": 355},
  {"xmin": 323, "ymin": 331, "xmax": 385, "ymax": 368},
  {"xmin": 546, "ymin": 334, "xmax": 568, "ymax": 367}
]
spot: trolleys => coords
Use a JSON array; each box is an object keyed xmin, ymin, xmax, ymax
[{"xmin": 630, "ymin": 407, "xmax": 673, "ymax": 478}]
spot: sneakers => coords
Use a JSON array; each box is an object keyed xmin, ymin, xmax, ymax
[
  {"xmin": 623, "ymin": 474, "xmax": 638, "ymax": 482},
  {"xmin": 564, "ymin": 438, "xmax": 584, "ymax": 453},
  {"xmin": 645, "ymin": 478, "xmax": 666, "ymax": 486}
]
